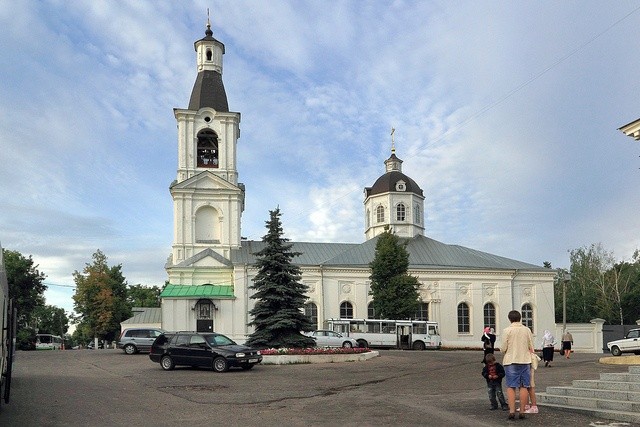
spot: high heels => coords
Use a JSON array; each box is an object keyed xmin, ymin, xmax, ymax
[
  {"xmin": 519, "ymin": 411, "xmax": 525, "ymax": 419},
  {"xmin": 509, "ymin": 411, "xmax": 515, "ymax": 421}
]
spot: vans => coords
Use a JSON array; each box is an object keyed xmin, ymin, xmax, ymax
[{"xmin": 149, "ymin": 331, "xmax": 263, "ymax": 373}]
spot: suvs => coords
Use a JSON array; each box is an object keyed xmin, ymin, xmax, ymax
[{"xmin": 119, "ymin": 328, "xmax": 170, "ymax": 355}]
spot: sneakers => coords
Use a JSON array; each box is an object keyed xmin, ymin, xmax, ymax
[
  {"xmin": 489, "ymin": 403, "xmax": 498, "ymax": 409},
  {"xmin": 502, "ymin": 404, "xmax": 508, "ymax": 410},
  {"xmin": 524, "ymin": 406, "xmax": 538, "ymax": 413},
  {"xmin": 516, "ymin": 404, "xmax": 530, "ymax": 412}
]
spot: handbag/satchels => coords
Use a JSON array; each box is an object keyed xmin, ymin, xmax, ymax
[
  {"xmin": 486, "ymin": 340, "xmax": 492, "ymax": 348},
  {"xmin": 560, "ymin": 344, "xmax": 564, "ymax": 355},
  {"xmin": 537, "ymin": 338, "xmax": 544, "ymax": 350}
]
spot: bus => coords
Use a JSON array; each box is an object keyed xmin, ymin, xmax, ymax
[
  {"xmin": 35, "ymin": 333, "xmax": 64, "ymax": 350},
  {"xmin": 323, "ymin": 318, "xmax": 441, "ymax": 350}
]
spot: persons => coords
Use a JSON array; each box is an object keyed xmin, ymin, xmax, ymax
[
  {"xmin": 479, "ymin": 328, "xmax": 496, "ymax": 363},
  {"xmin": 500, "ymin": 310, "xmax": 533, "ymax": 419},
  {"xmin": 516, "ymin": 347, "xmax": 541, "ymax": 414},
  {"xmin": 485, "ymin": 353, "xmax": 510, "ymax": 411},
  {"xmin": 541, "ymin": 330, "xmax": 557, "ymax": 368},
  {"xmin": 561, "ymin": 329, "xmax": 573, "ymax": 360},
  {"xmin": 482, "ymin": 327, "xmax": 490, "ymax": 351}
]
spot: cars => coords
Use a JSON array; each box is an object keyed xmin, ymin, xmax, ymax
[
  {"xmin": 305, "ymin": 330, "xmax": 359, "ymax": 349},
  {"xmin": 607, "ymin": 328, "xmax": 640, "ymax": 356},
  {"xmin": 86, "ymin": 340, "xmax": 103, "ymax": 349}
]
